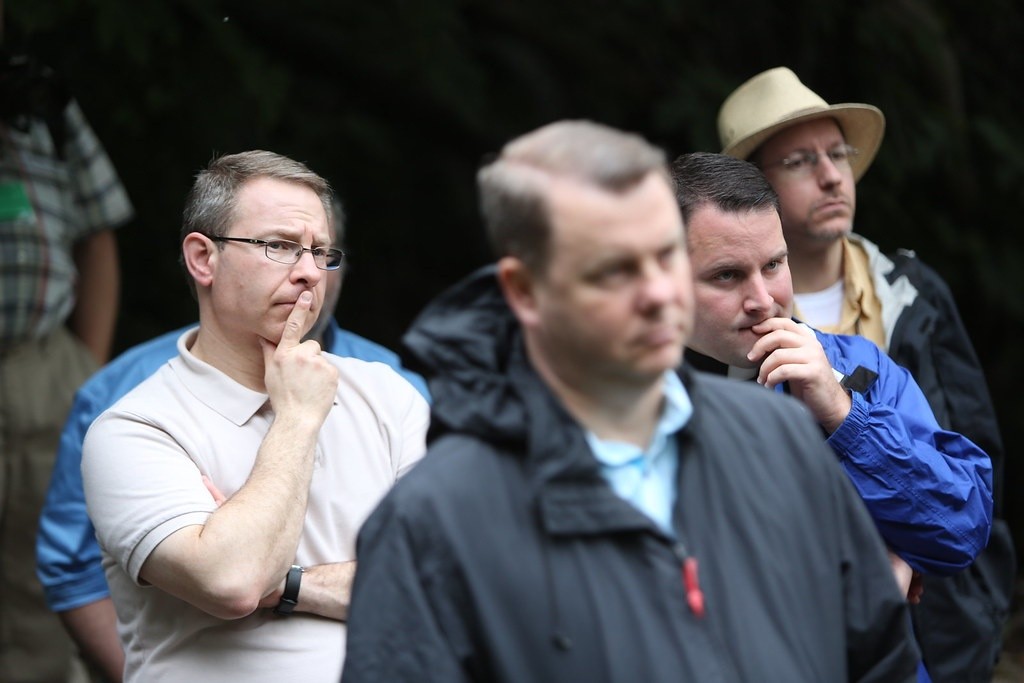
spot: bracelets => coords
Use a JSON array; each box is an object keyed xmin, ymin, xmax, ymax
[{"xmin": 272, "ymin": 564, "xmax": 305, "ymax": 616}]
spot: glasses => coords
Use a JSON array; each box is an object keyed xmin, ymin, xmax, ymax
[
  {"xmin": 760, "ymin": 145, "xmax": 859, "ymax": 174},
  {"xmin": 204, "ymin": 234, "xmax": 346, "ymax": 269}
]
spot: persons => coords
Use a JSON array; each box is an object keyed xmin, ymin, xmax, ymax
[
  {"xmin": 30, "ymin": 63, "xmax": 436, "ymax": 683},
  {"xmin": 0, "ymin": 0, "xmax": 135, "ymax": 683},
  {"xmin": 339, "ymin": 66, "xmax": 1016, "ymax": 683}
]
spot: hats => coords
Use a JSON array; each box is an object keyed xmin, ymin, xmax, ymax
[{"xmin": 716, "ymin": 65, "xmax": 885, "ymax": 183}]
